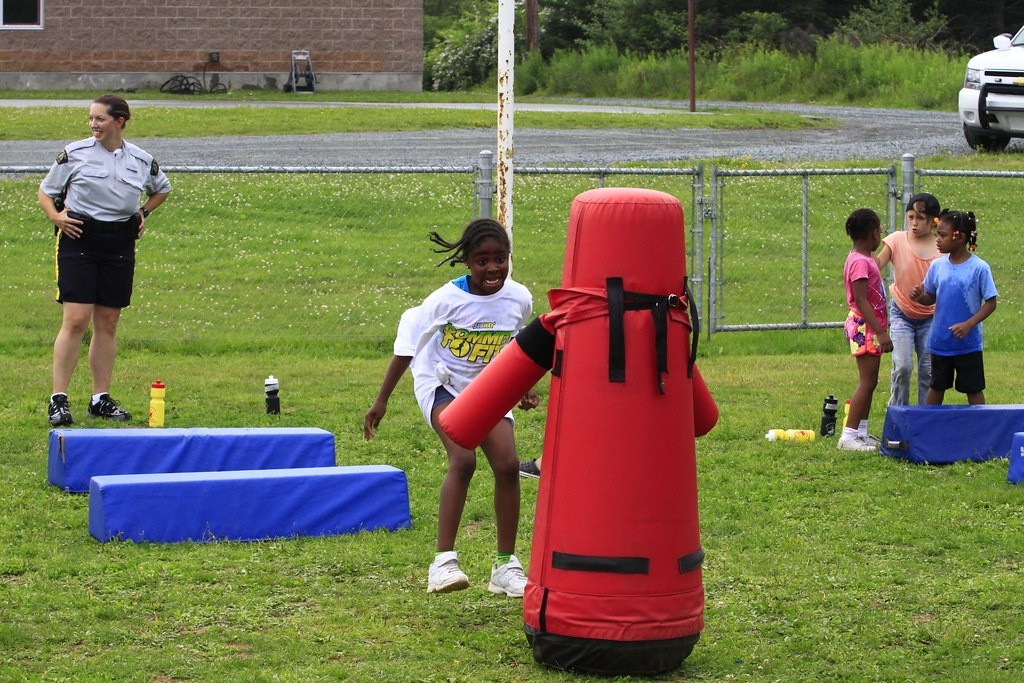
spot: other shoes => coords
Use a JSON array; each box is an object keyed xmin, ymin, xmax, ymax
[{"xmin": 519, "ymin": 458, "xmax": 540, "ymax": 479}]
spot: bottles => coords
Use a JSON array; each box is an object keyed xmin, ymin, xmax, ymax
[
  {"xmin": 264, "ymin": 374, "xmax": 279, "ymax": 415},
  {"xmin": 819, "ymin": 395, "xmax": 838, "ymax": 437},
  {"xmin": 843, "ymin": 400, "xmax": 851, "ymax": 430},
  {"xmin": 765, "ymin": 428, "xmax": 814, "ymax": 443},
  {"xmin": 149, "ymin": 380, "xmax": 166, "ymax": 426}
]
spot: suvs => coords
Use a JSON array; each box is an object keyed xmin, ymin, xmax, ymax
[{"xmin": 955, "ymin": 23, "xmax": 1024, "ymax": 154}]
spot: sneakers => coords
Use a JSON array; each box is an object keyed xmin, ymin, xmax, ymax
[
  {"xmin": 837, "ymin": 435, "xmax": 876, "ymax": 451},
  {"xmin": 489, "ymin": 555, "xmax": 528, "ymax": 598},
  {"xmin": 428, "ymin": 552, "xmax": 470, "ymax": 593},
  {"xmin": 858, "ymin": 434, "xmax": 882, "ymax": 446},
  {"xmin": 86, "ymin": 393, "xmax": 132, "ymax": 422},
  {"xmin": 48, "ymin": 393, "xmax": 74, "ymax": 426}
]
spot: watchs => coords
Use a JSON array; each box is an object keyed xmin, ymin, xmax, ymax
[{"xmin": 140, "ymin": 206, "xmax": 149, "ymax": 218}]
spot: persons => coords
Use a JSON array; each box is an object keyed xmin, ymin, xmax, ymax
[
  {"xmin": 837, "ymin": 210, "xmax": 894, "ymax": 450},
  {"xmin": 519, "ymin": 457, "xmax": 541, "ymax": 479},
  {"xmin": 37, "ymin": 95, "xmax": 171, "ymax": 427},
  {"xmin": 364, "ymin": 219, "xmax": 539, "ymax": 597},
  {"xmin": 909, "ymin": 211, "xmax": 998, "ymax": 405},
  {"xmin": 871, "ymin": 194, "xmax": 947, "ymax": 406}
]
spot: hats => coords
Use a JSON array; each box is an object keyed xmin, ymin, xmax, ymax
[{"xmin": 906, "ymin": 193, "xmax": 940, "ymax": 218}]
